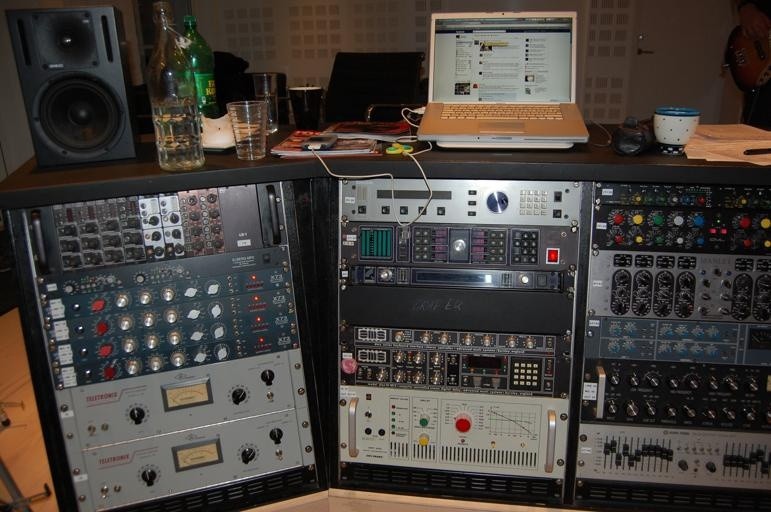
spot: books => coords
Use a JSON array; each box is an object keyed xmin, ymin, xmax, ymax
[{"xmin": 270, "ymin": 118, "xmax": 410, "ymax": 156}]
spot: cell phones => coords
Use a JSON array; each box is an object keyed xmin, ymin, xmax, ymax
[{"xmin": 300, "ymin": 134, "xmax": 338, "ymax": 150}]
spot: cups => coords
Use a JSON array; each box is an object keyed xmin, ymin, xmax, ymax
[
  {"xmin": 653, "ymin": 107, "xmax": 701, "ymax": 157},
  {"xmin": 225, "ymin": 101, "xmax": 269, "ymax": 160},
  {"xmin": 253, "ymin": 72, "xmax": 279, "ymax": 135},
  {"xmin": 288, "ymin": 85, "xmax": 323, "ymax": 133}
]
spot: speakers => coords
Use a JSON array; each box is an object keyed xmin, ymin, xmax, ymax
[{"xmin": 5, "ymin": 6, "xmax": 140, "ymax": 169}]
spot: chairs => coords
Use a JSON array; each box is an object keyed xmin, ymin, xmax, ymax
[{"xmin": 320, "ymin": 50, "xmax": 426, "ymax": 132}]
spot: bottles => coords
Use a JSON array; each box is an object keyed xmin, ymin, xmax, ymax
[
  {"xmin": 182, "ymin": 15, "xmax": 217, "ymax": 118},
  {"xmin": 611, "ymin": 115, "xmax": 651, "ymax": 154},
  {"xmin": 145, "ymin": 5, "xmax": 206, "ymax": 173}
]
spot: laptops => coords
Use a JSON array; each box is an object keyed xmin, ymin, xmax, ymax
[{"xmin": 414, "ymin": 11, "xmax": 592, "ymax": 145}]
